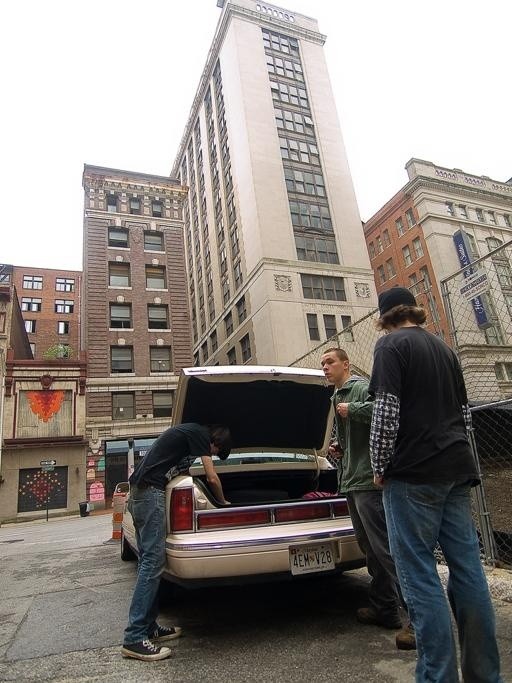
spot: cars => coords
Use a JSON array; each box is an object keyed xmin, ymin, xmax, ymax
[{"xmin": 120, "ymin": 365, "xmax": 367, "ymax": 607}]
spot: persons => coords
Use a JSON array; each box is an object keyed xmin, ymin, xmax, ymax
[
  {"xmin": 320, "ymin": 348, "xmax": 416, "ymax": 650},
  {"xmin": 369, "ymin": 288, "xmax": 505, "ymax": 683},
  {"xmin": 121, "ymin": 423, "xmax": 232, "ymax": 662}
]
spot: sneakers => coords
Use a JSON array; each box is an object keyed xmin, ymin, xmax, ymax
[
  {"xmin": 148, "ymin": 624, "xmax": 183, "ymax": 644},
  {"xmin": 357, "ymin": 607, "xmax": 402, "ymax": 629},
  {"xmin": 121, "ymin": 639, "xmax": 171, "ymax": 661},
  {"xmin": 396, "ymin": 623, "xmax": 416, "ymax": 651}
]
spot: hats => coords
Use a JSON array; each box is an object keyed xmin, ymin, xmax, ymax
[{"xmin": 378, "ymin": 283, "xmax": 417, "ymax": 315}]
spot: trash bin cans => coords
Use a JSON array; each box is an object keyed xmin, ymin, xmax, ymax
[{"xmin": 78, "ymin": 500, "xmax": 90, "ymax": 517}]
